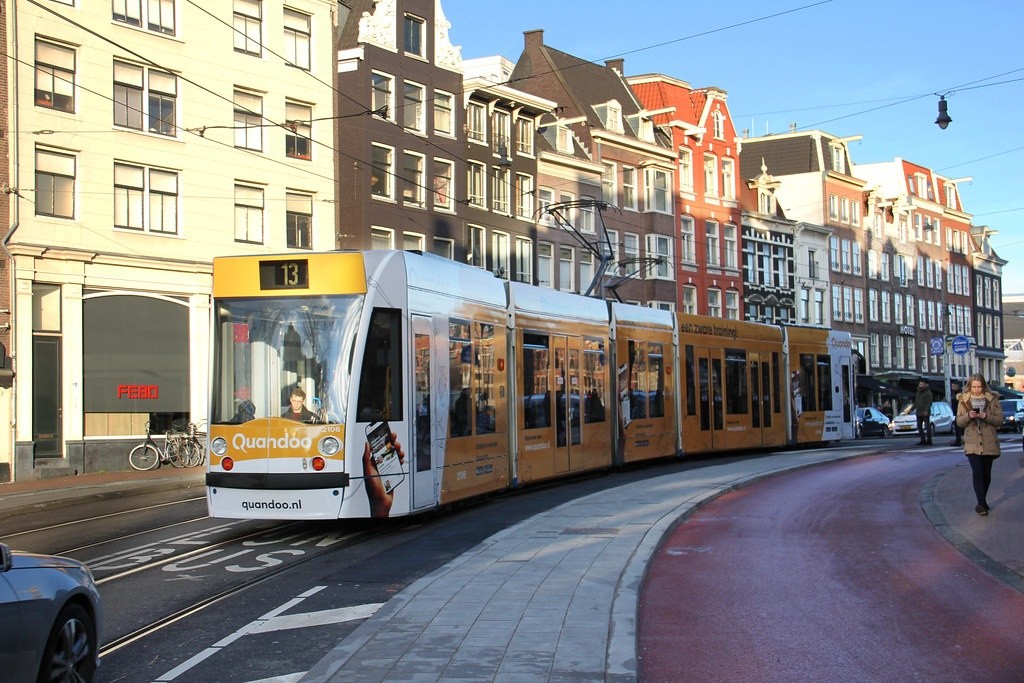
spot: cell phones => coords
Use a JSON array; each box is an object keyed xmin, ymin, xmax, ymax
[
  {"xmin": 972, "ymin": 408, "xmax": 979, "ymax": 413},
  {"xmin": 791, "ymin": 368, "xmax": 803, "ymax": 416},
  {"xmin": 367, "ymin": 421, "xmax": 405, "ymax": 494},
  {"xmin": 618, "ymin": 362, "xmax": 632, "ymax": 427}
]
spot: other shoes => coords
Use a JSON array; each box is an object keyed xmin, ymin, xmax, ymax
[
  {"xmin": 926, "ymin": 441, "xmax": 932, "ymax": 445},
  {"xmin": 916, "ymin": 441, "xmax": 926, "ymax": 445},
  {"xmin": 976, "ymin": 504, "xmax": 989, "ymax": 512},
  {"xmin": 978, "ymin": 506, "xmax": 987, "ymax": 514},
  {"xmin": 950, "ymin": 442, "xmax": 961, "ymax": 447}
]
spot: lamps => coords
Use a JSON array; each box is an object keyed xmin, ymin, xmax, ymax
[
  {"xmin": 497, "ymin": 143, "xmax": 512, "ymax": 173},
  {"xmin": 923, "ymin": 222, "xmax": 934, "ymax": 232},
  {"xmin": 934, "ymin": 95, "xmax": 953, "ymax": 130}
]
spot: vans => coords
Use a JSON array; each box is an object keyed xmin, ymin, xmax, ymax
[{"xmin": 995, "ymin": 399, "xmax": 1024, "ymax": 433}]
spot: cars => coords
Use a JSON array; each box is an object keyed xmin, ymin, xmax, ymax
[
  {"xmin": 887, "ymin": 402, "xmax": 957, "ymax": 437},
  {"xmin": 855, "ymin": 406, "xmax": 891, "ymax": 439},
  {"xmin": 0, "ymin": 541, "xmax": 102, "ymax": 683}
]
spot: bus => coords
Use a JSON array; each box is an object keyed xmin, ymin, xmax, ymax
[{"xmin": 204, "ymin": 199, "xmax": 870, "ymax": 523}]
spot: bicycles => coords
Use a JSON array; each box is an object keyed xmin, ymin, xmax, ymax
[{"xmin": 128, "ymin": 418, "xmax": 207, "ymax": 471}]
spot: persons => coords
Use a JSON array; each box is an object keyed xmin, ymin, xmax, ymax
[
  {"xmin": 283, "ymin": 388, "xmax": 320, "ymax": 421},
  {"xmin": 951, "ymin": 381, "xmax": 965, "ymax": 447},
  {"xmin": 229, "ymin": 400, "xmax": 256, "ymax": 422},
  {"xmin": 909, "ymin": 381, "xmax": 933, "ymax": 445},
  {"xmin": 362, "ymin": 432, "xmax": 405, "ymax": 517},
  {"xmin": 956, "ymin": 374, "xmax": 1003, "ymax": 516},
  {"xmin": 878, "ymin": 399, "xmax": 893, "ymax": 423}
]
